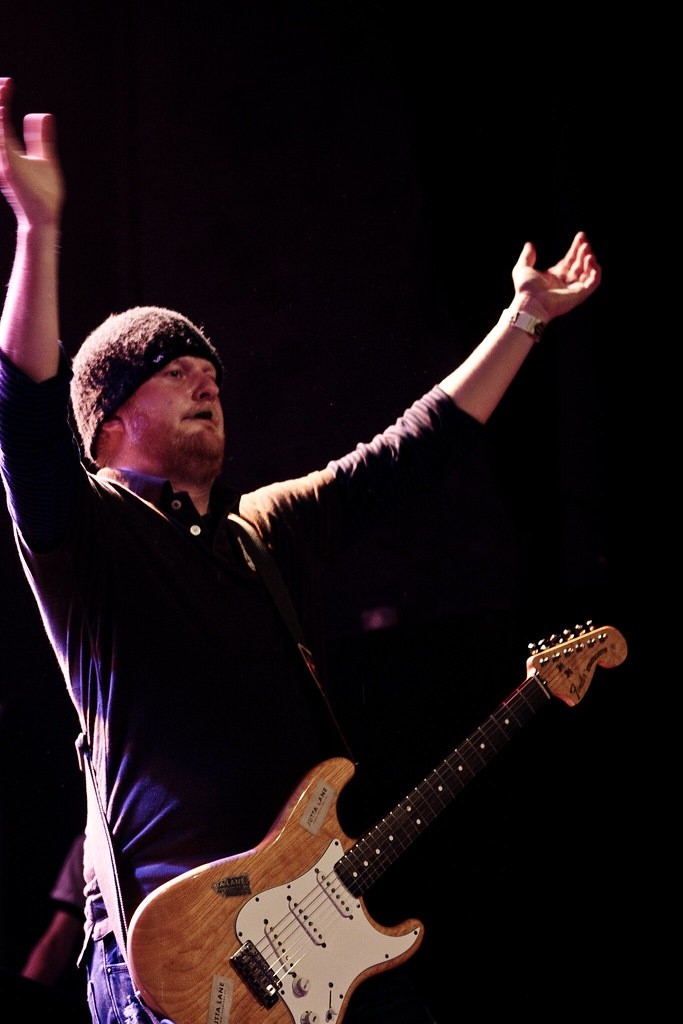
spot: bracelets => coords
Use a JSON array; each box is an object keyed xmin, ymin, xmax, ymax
[{"xmin": 498, "ymin": 307, "xmax": 548, "ymax": 346}]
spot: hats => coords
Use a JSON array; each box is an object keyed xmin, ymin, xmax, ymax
[{"xmin": 67, "ymin": 304, "xmax": 226, "ymax": 466}]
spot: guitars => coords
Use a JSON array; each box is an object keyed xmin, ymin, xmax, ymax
[{"xmin": 124, "ymin": 622, "xmax": 630, "ymax": 1023}]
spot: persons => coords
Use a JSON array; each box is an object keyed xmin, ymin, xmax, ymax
[
  {"xmin": 23, "ymin": 831, "xmax": 85, "ymax": 984},
  {"xmin": 0, "ymin": 76, "xmax": 601, "ymax": 1024}
]
